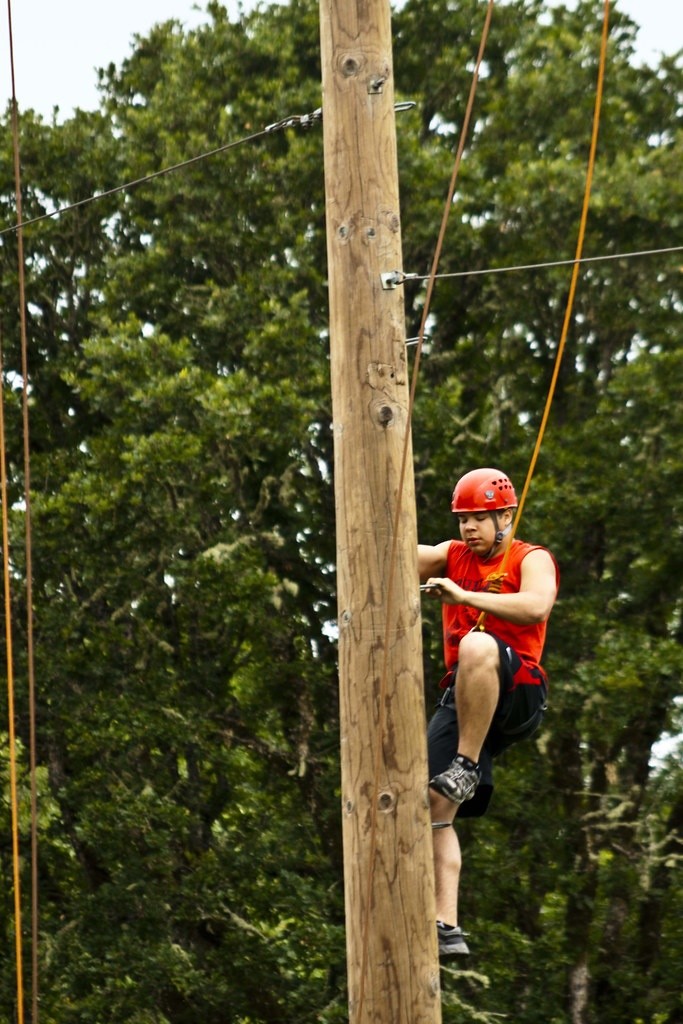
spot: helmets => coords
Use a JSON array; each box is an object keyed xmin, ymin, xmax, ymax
[{"xmin": 450, "ymin": 467, "xmax": 518, "ymax": 513}]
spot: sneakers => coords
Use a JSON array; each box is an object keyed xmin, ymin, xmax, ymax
[
  {"xmin": 428, "ymin": 753, "xmax": 482, "ymax": 805},
  {"xmin": 435, "ymin": 917, "xmax": 470, "ymax": 962}
]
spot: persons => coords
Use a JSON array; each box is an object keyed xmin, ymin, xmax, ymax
[{"xmin": 418, "ymin": 469, "xmax": 558, "ymax": 958}]
